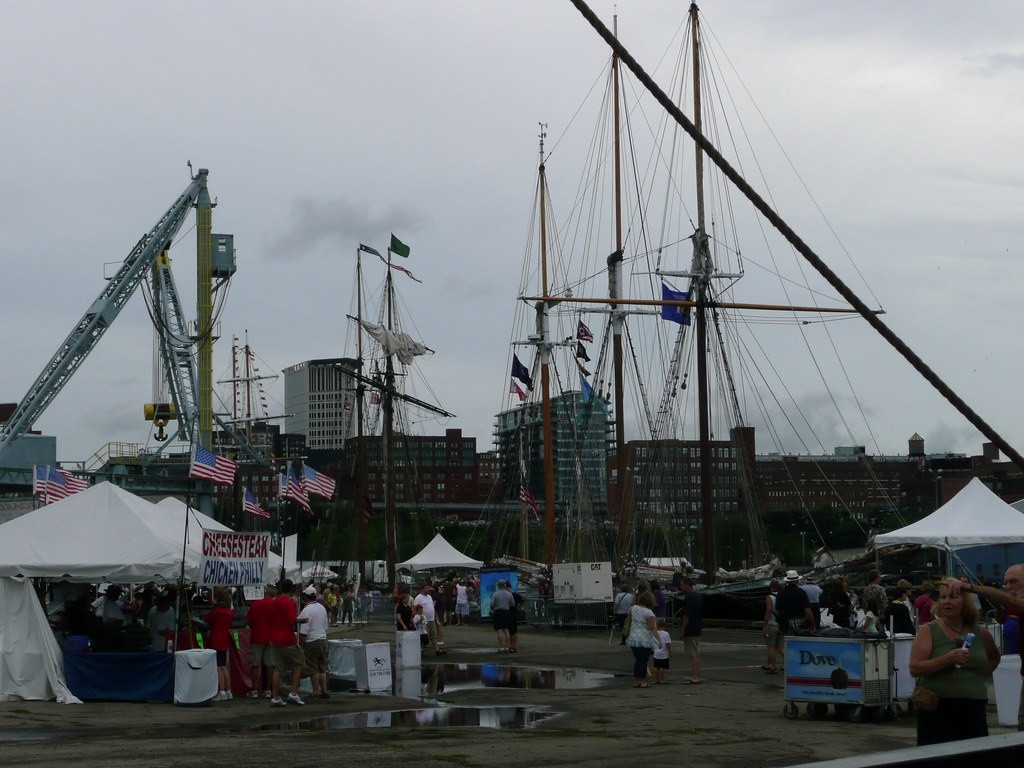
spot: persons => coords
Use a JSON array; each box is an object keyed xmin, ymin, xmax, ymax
[{"xmin": 0, "ymin": 561, "xmax": 1024, "ymax": 768}]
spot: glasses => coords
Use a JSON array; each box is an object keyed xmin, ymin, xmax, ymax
[{"xmin": 1002, "ymin": 578, "xmax": 1024, "ymax": 588}]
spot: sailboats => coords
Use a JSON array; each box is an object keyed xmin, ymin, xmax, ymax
[{"xmin": 85, "ymin": 0, "xmax": 944, "ymax": 623}]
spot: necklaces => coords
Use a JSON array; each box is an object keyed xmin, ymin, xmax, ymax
[{"xmin": 943, "ymin": 617, "xmax": 965, "ymax": 642}]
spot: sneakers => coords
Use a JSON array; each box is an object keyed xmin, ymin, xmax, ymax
[
  {"xmin": 211, "ymin": 692, "xmax": 227, "ymax": 701},
  {"xmin": 262, "ymin": 690, "xmax": 271, "ymax": 697},
  {"xmin": 225, "ymin": 691, "xmax": 233, "ymax": 699},
  {"xmin": 288, "ymin": 692, "xmax": 305, "ymax": 704},
  {"xmin": 271, "ymin": 696, "xmax": 287, "ymax": 706},
  {"xmin": 246, "ymin": 690, "xmax": 258, "ymax": 698}
]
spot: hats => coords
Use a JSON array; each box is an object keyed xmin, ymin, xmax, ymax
[
  {"xmin": 302, "ymin": 583, "xmax": 316, "ymax": 595},
  {"xmin": 621, "ymin": 584, "xmax": 629, "ymax": 592},
  {"xmin": 784, "ymin": 570, "xmax": 803, "ymax": 582},
  {"xmin": 497, "ymin": 579, "xmax": 505, "ymax": 586},
  {"xmin": 804, "ymin": 577, "xmax": 815, "ymax": 584}
]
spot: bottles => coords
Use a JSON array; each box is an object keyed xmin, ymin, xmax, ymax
[{"xmin": 421, "ymin": 612, "xmax": 425, "ymax": 624}]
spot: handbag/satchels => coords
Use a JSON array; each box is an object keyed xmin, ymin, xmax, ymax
[
  {"xmin": 910, "ymin": 686, "xmax": 941, "ymax": 711},
  {"xmin": 621, "ymin": 610, "xmax": 632, "ymax": 635}
]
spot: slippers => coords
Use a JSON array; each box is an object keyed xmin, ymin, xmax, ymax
[
  {"xmin": 307, "ymin": 693, "xmax": 323, "ymax": 699},
  {"xmin": 641, "ymin": 683, "xmax": 652, "ymax": 688},
  {"xmin": 634, "ymin": 683, "xmax": 641, "ymax": 687},
  {"xmin": 682, "ymin": 679, "xmax": 703, "ymax": 684},
  {"xmin": 323, "ymin": 693, "xmax": 330, "ymax": 700}
]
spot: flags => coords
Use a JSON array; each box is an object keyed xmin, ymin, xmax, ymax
[
  {"xmin": 241, "ymin": 487, "xmax": 270, "ymax": 518},
  {"xmin": 661, "ymin": 282, "xmax": 692, "ymax": 327},
  {"xmin": 370, "ymin": 393, "xmax": 379, "ymax": 405},
  {"xmin": 510, "ymin": 380, "xmax": 528, "ymax": 401},
  {"xmin": 519, "ymin": 463, "xmax": 541, "ymax": 526},
  {"xmin": 511, "ymin": 354, "xmax": 534, "ymax": 393},
  {"xmin": 390, "ymin": 233, "xmax": 410, "ymax": 258},
  {"xmin": 575, "ymin": 322, "xmax": 594, "ymax": 343},
  {"xmin": 189, "ymin": 444, "xmax": 238, "ymax": 485},
  {"xmin": 32, "ymin": 463, "xmax": 87, "ymax": 505},
  {"xmin": 575, "ymin": 340, "xmax": 591, "ymax": 362},
  {"xmin": 278, "ymin": 465, "xmax": 334, "ymax": 516}
]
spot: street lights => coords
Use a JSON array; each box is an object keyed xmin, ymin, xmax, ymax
[{"xmin": 928, "ymin": 468, "xmax": 943, "ymax": 568}]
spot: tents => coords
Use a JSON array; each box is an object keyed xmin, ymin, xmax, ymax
[
  {"xmin": 0, "ymin": 480, "xmax": 300, "ymax": 702},
  {"xmin": 874, "ymin": 478, "xmax": 1024, "ymax": 581},
  {"xmin": 394, "ymin": 531, "xmax": 485, "ymax": 599}
]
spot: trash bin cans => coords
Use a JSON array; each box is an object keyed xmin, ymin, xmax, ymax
[
  {"xmin": 327, "ymin": 638, "xmax": 362, "ymax": 691},
  {"xmin": 992, "ymin": 654, "xmax": 1022, "ymax": 726},
  {"xmin": 174, "ymin": 649, "xmax": 218, "ymax": 706}
]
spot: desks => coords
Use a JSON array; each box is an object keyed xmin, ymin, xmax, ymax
[
  {"xmin": 354, "ymin": 712, "xmax": 392, "ymax": 728},
  {"xmin": 396, "ymin": 630, "xmax": 421, "ymax": 669},
  {"xmin": 62, "ymin": 652, "xmax": 175, "ymax": 703},
  {"xmin": 992, "ymin": 654, "xmax": 1023, "ymax": 726},
  {"xmin": 353, "ymin": 641, "xmax": 393, "ymax": 692},
  {"xmin": 328, "ymin": 640, "xmax": 363, "ymax": 680},
  {"xmin": 173, "ymin": 649, "xmax": 219, "ymax": 707},
  {"xmin": 396, "ymin": 667, "xmax": 421, "ymax": 701}
]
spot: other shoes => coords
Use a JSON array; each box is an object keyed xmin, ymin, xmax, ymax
[
  {"xmin": 498, "ymin": 646, "xmax": 518, "ymax": 653},
  {"xmin": 436, "ymin": 649, "xmax": 448, "ymax": 655},
  {"xmin": 329, "ymin": 622, "xmax": 351, "ymax": 627},
  {"xmin": 760, "ymin": 664, "xmax": 777, "ymax": 672}
]
